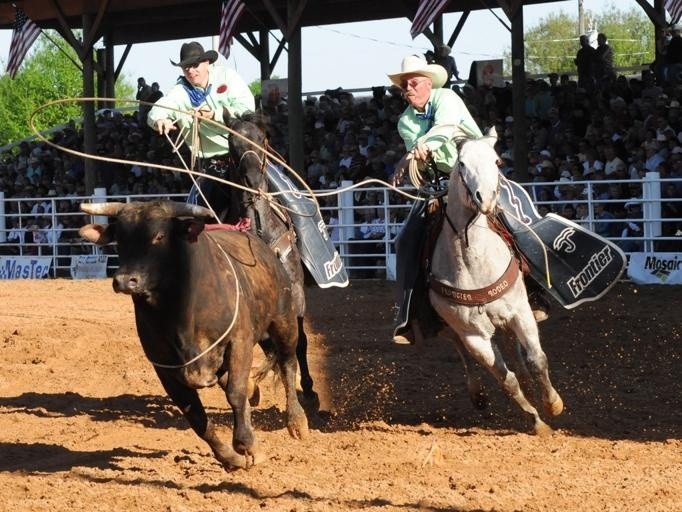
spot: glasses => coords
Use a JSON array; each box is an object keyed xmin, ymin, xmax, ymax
[
  {"xmin": 186, "ymin": 63, "xmax": 199, "ymax": 68},
  {"xmin": 401, "ymin": 79, "xmax": 419, "ymax": 88}
]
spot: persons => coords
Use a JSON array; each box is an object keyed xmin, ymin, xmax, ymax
[
  {"xmin": 144, "ymin": 42, "xmax": 321, "ymax": 290},
  {"xmin": 385, "ymin": 55, "xmax": 552, "ymax": 346}
]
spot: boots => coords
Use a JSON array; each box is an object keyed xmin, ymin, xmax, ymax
[
  {"xmin": 525, "ymin": 277, "xmax": 550, "ymax": 321},
  {"xmin": 394, "ymin": 289, "xmax": 415, "ymax": 344}
]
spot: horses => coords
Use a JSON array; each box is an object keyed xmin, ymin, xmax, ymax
[
  {"xmin": 422, "ymin": 123, "xmax": 566, "ymax": 437},
  {"xmin": 195, "ymin": 107, "xmax": 321, "ymax": 414}
]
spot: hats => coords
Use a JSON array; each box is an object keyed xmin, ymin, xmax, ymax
[
  {"xmin": 387, "ymin": 56, "xmax": 448, "ymax": 88},
  {"xmin": 539, "ymin": 150, "xmax": 551, "ymax": 158},
  {"xmin": 624, "ymin": 197, "xmax": 643, "ymax": 209},
  {"xmin": 554, "ymin": 170, "xmax": 572, "ymax": 185},
  {"xmin": 540, "ymin": 160, "xmax": 554, "ymax": 168},
  {"xmin": 170, "ymin": 42, "xmax": 218, "ymax": 67}
]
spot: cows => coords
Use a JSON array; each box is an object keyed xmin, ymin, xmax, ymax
[{"xmin": 74, "ymin": 195, "xmax": 310, "ymax": 474}]
